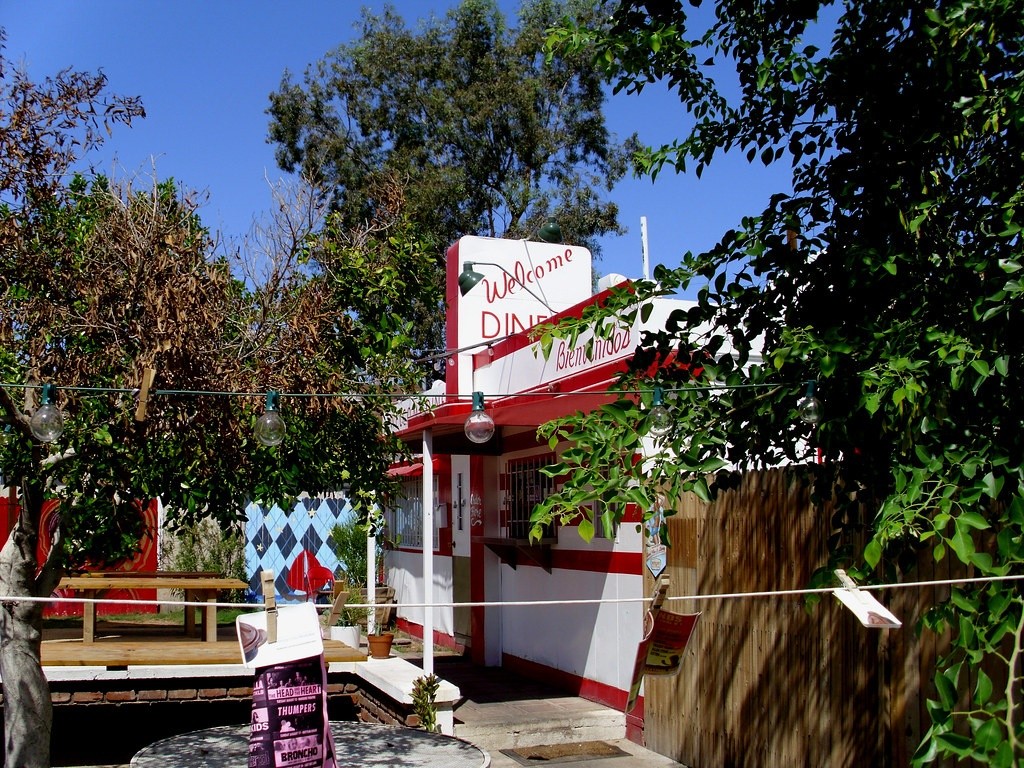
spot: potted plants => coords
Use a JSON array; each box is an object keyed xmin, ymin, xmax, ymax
[
  {"xmin": 368, "ymin": 622, "xmax": 394, "ymax": 659},
  {"xmin": 322, "ymin": 577, "xmax": 375, "ymax": 651},
  {"xmin": 329, "ymin": 514, "xmax": 395, "ymax": 629}
]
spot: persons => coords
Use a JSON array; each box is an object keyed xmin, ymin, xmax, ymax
[
  {"xmin": 267, "ymin": 671, "xmax": 309, "ymax": 689},
  {"xmin": 252, "ymin": 712, "xmax": 259, "ymax": 723},
  {"xmin": 275, "ymin": 738, "xmax": 309, "ymax": 752},
  {"xmin": 280, "ymin": 716, "xmax": 309, "ymax": 733}
]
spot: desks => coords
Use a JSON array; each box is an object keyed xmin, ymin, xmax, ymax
[
  {"xmin": 39, "ymin": 640, "xmax": 368, "ymax": 665},
  {"xmin": 56, "ymin": 571, "xmax": 248, "ymax": 642},
  {"xmin": 129, "ymin": 720, "xmax": 492, "ymax": 768}
]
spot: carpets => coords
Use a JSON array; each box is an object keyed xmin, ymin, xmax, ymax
[{"xmin": 499, "ymin": 740, "xmax": 634, "ymax": 766}]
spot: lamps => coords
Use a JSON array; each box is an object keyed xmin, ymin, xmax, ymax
[
  {"xmin": 523, "ymin": 217, "xmax": 562, "ymax": 317},
  {"xmin": 458, "ymin": 261, "xmax": 560, "ymax": 314}
]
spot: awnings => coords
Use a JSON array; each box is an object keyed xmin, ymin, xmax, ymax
[{"xmin": 384, "ymin": 459, "xmax": 440, "ymax": 476}]
